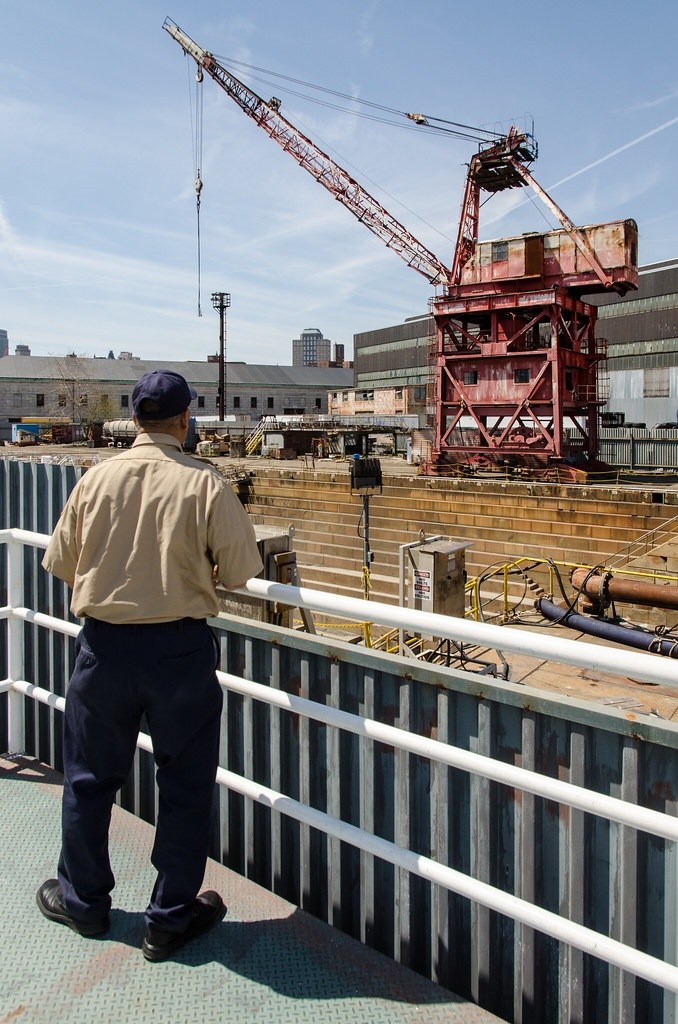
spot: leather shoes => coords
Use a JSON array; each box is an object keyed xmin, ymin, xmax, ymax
[
  {"xmin": 142, "ymin": 890, "xmax": 225, "ymax": 961},
  {"xmin": 35, "ymin": 878, "xmax": 112, "ymax": 936}
]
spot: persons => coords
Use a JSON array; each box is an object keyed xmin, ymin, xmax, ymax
[{"xmin": 36, "ymin": 368, "xmax": 265, "ymax": 964}]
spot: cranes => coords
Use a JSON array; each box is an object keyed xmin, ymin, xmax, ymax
[{"xmin": 163, "ymin": 18, "xmax": 643, "ymax": 481}]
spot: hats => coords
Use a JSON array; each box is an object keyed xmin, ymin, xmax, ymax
[{"xmin": 131, "ymin": 369, "xmax": 198, "ymax": 419}]
